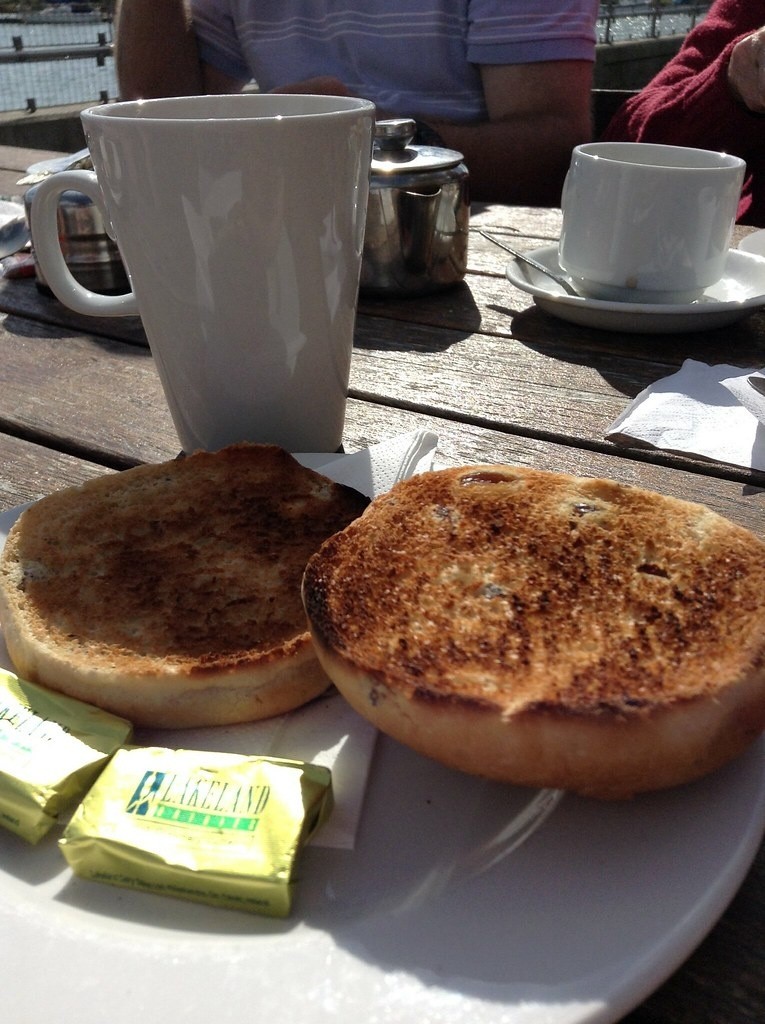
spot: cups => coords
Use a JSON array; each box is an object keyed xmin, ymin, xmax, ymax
[
  {"xmin": 24, "ymin": 169, "xmax": 127, "ymax": 298},
  {"xmin": 30, "ymin": 94, "xmax": 376, "ymax": 456},
  {"xmin": 558, "ymin": 142, "xmax": 746, "ymax": 305},
  {"xmin": 360, "ymin": 118, "xmax": 469, "ymax": 289}
]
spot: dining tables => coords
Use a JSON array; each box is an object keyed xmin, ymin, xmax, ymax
[{"xmin": 0, "ymin": 145, "xmax": 765, "ymax": 1024}]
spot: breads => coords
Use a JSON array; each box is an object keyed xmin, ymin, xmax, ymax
[
  {"xmin": 301, "ymin": 463, "xmax": 765, "ymax": 799},
  {"xmin": 0, "ymin": 439, "xmax": 375, "ymax": 731}
]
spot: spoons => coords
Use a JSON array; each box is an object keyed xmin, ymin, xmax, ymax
[{"xmin": 26, "ymin": 147, "xmax": 90, "ymax": 173}]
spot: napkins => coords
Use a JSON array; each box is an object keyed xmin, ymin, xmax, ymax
[
  {"xmin": 289, "ymin": 426, "xmax": 439, "ymax": 502},
  {"xmin": 603, "ymin": 358, "xmax": 765, "ymax": 473}
]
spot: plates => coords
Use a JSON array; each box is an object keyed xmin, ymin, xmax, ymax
[
  {"xmin": 506, "ymin": 244, "xmax": 765, "ymax": 334},
  {"xmin": 0, "ymin": 500, "xmax": 765, "ymax": 1024}
]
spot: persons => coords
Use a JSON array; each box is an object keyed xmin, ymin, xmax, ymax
[
  {"xmin": 602, "ymin": 0, "xmax": 764, "ymax": 227},
  {"xmin": 115, "ymin": 0, "xmax": 599, "ymax": 207}
]
